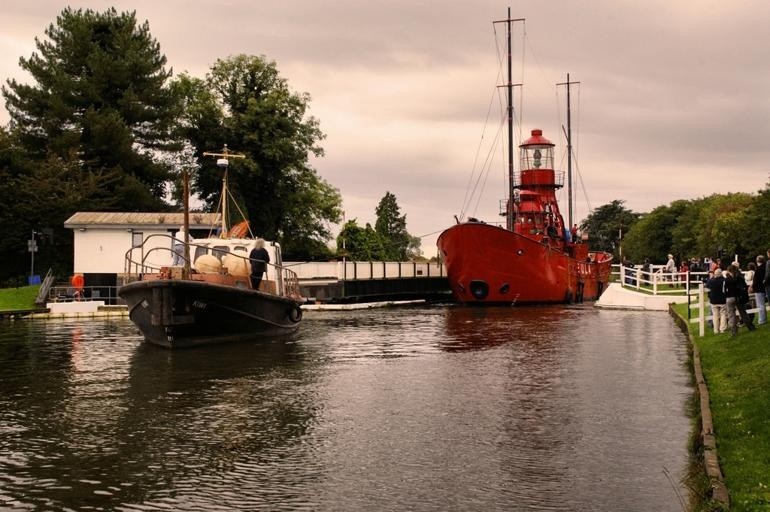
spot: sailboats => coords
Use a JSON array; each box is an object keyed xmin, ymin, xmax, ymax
[{"xmin": 435, "ymin": 5, "xmax": 617, "ymax": 308}]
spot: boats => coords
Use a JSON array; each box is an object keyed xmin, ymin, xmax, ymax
[{"xmin": 110, "ymin": 141, "xmax": 309, "ymax": 346}]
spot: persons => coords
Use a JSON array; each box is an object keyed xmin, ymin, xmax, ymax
[
  {"xmin": 249, "ymin": 238, "xmax": 270, "ymax": 291},
  {"xmin": 571, "ymin": 224, "xmax": 577, "ymax": 243},
  {"xmin": 622, "ymin": 256, "xmax": 635, "ymax": 285},
  {"xmin": 666, "ymin": 248, "xmax": 770, "ymax": 336},
  {"xmin": 642, "ymin": 258, "xmax": 650, "ymax": 286}
]
